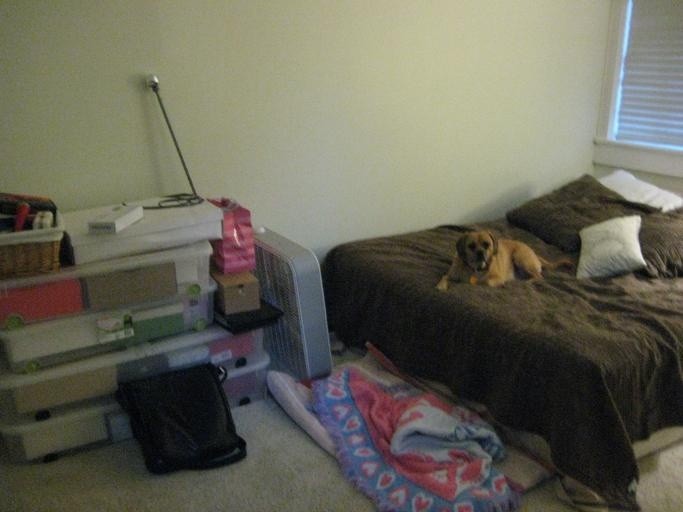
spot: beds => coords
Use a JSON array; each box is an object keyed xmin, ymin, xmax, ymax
[{"xmin": 321, "ymin": 169, "xmax": 683, "ymax": 512}]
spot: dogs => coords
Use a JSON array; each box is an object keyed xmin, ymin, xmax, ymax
[{"xmin": 435, "ymin": 229, "xmax": 575, "ymax": 292}]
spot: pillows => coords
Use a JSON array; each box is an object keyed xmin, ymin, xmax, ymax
[
  {"xmin": 596, "ymin": 169, "xmax": 683, "ymax": 213},
  {"xmin": 505, "ymin": 174, "xmax": 682, "ymax": 279},
  {"xmin": 576, "ymin": 215, "xmax": 647, "ymax": 280}
]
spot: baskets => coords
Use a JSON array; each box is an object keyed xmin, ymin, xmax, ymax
[{"xmin": 0, "ymin": 209, "xmax": 66, "ymax": 280}]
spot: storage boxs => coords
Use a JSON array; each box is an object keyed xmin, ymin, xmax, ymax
[{"xmin": 0, "ymin": 243, "xmax": 270, "ymax": 466}]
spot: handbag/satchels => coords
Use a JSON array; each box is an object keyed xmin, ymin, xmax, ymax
[{"xmin": 114, "ymin": 360, "xmax": 248, "ymax": 475}]
